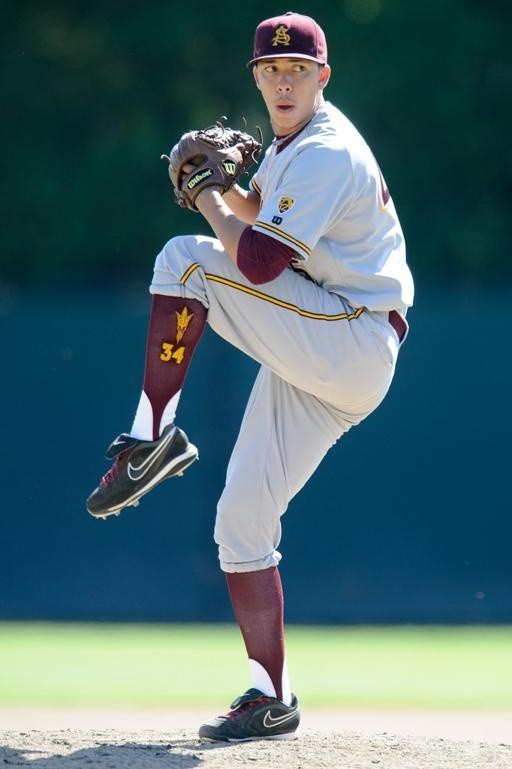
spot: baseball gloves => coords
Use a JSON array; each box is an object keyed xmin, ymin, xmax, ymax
[{"xmin": 169, "ymin": 127, "xmax": 262, "ymax": 214}]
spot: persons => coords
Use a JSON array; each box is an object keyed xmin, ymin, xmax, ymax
[{"xmin": 83, "ymin": 10, "xmax": 418, "ymax": 744}]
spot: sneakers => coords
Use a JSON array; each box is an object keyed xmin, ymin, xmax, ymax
[
  {"xmin": 85, "ymin": 422, "xmax": 201, "ymax": 522},
  {"xmin": 198, "ymin": 687, "xmax": 301, "ymax": 745}
]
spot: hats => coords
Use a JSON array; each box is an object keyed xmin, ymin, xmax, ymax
[{"xmin": 245, "ymin": 10, "xmax": 329, "ymax": 69}]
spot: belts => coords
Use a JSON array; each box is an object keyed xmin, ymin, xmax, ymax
[{"xmin": 387, "ymin": 308, "xmax": 409, "ymax": 344}]
